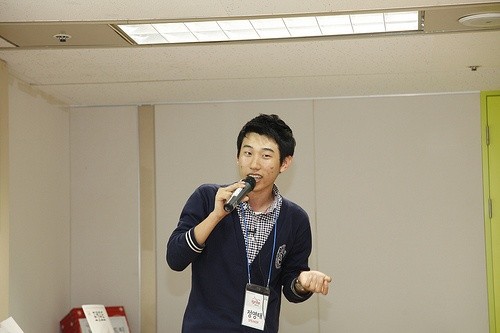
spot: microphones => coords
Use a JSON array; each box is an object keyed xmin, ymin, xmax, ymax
[{"xmin": 224, "ymin": 176, "xmax": 256, "ymax": 212}]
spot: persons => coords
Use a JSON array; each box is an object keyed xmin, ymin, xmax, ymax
[{"xmin": 166, "ymin": 113, "xmax": 332, "ymax": 333}]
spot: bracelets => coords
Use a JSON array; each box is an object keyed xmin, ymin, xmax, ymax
[{"xmin": 296, "ymin": 278, "xmax": 309, "ymax": 293}]
